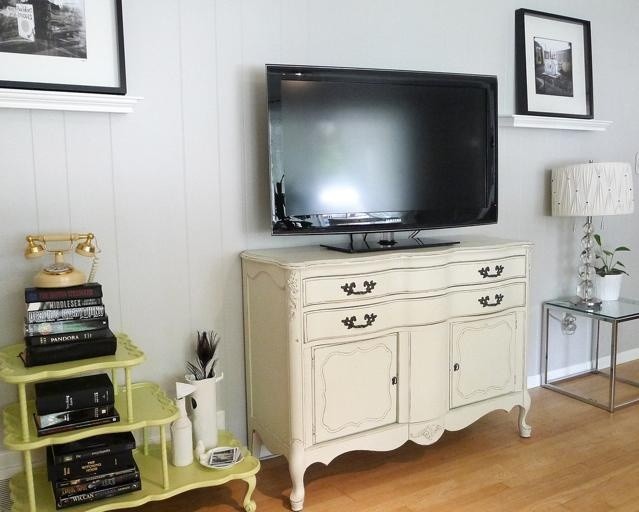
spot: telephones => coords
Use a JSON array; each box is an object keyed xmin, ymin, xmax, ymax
[{"xmin": 23, "ymin": 230, "xmax": 104, "ymax": 288}]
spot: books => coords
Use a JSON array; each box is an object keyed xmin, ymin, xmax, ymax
[{"xmin": 18, "ymin": 281, "xmax": 142, "ymax": 511}]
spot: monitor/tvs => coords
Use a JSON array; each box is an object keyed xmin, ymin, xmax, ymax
[{"xmin": 266, "ymin": 63, "xmax": 499, "ymax": 254}]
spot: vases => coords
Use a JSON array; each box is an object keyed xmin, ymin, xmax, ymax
[{"xmin": 182, "ymin": 366, "xmax": 222, "ymax": 452}]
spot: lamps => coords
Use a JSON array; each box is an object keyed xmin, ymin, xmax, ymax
[{"xmin": 545, "ymin": 156, "xmax": 636, "ymax": 314}]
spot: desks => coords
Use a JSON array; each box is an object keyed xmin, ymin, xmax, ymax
[{"xmin": 537, "ymin": 293, "xmax": 639, "ymax": 415}]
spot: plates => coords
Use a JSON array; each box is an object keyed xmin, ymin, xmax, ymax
[{"xmin": 199, "ymin": 446, "xmax": 243, "ymax": 471}]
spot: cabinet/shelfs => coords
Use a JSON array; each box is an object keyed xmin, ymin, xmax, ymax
[
  {"xmin": 237, "ymin": 233, "xmax": 542, "ymax": 512},
  {"xmin": 0, "ymin": 328, "xmax": 262, "ymax": 511}
]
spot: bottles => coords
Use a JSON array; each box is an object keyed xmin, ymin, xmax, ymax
[{"xmin": 170, "ymin": 397, "xmax": 194, "ymax": 467}]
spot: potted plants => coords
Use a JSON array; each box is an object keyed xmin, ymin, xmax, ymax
[{"xmin": 587, "ymin": 230, "xmax": 633, "ymax": 302}]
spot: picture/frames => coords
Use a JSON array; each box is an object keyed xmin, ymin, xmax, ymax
[
  {"xmin": 512, "ymin": 4, "xmax": 598, "ymax": 122},
  {"xmin": 0, "ymin": 1, "xmax": 132, "ymax": 96}
]
